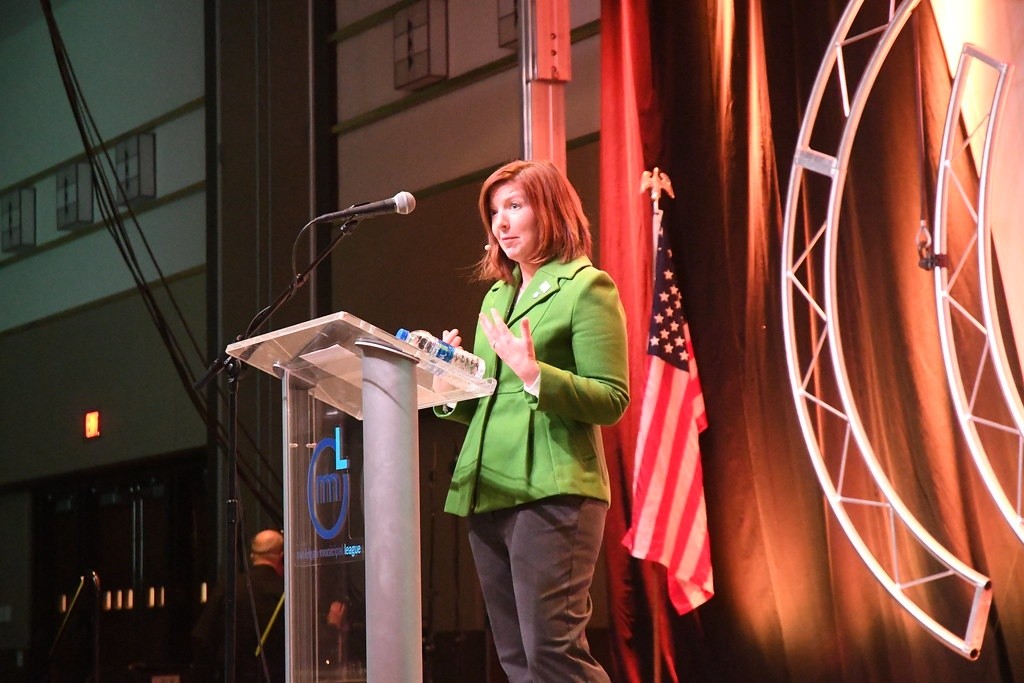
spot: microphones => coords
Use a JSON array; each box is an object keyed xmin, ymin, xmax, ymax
[
  {"xmin": 485, "ymin": 245, "xmax": 492, "ymax": 250},
  {"xmin": 314, "ymin": 190, "xmax": 416, "ymax": 225}
]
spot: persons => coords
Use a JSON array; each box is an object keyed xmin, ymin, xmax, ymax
[
  {"xmin": 191, "ymin": 529, "xmax": 347, "ymax": 683},
  {"xmin": 435, "ymin": 159, "xmax": 630, "ymax": 682}
]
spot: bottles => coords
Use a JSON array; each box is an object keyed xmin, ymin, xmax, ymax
[{"xmin": 397, "ymin": 327, "xmax": 486, "ymax": 378}]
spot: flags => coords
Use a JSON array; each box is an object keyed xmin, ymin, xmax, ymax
[{"xmin": 620, "ymin": 210, "xmax": 715, "ymax": 618}]
